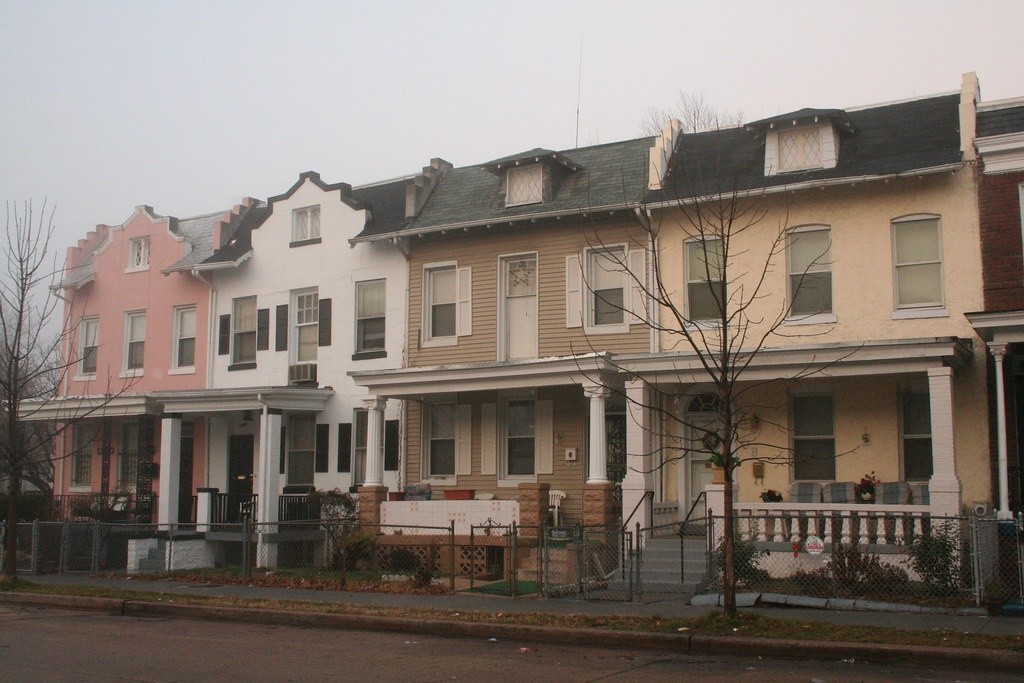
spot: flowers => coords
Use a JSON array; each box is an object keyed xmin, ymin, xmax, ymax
[
  {"xmin": 759, "ymin": 489, "xmax": 784, "ymax": 503},
  {"xmin": 857, "ymin": 469, "xmax": 880, "ymax": 492}
]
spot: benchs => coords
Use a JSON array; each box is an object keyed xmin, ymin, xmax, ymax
[{"xmin": 84, "ymin": 493, "xmax": 130, "ymax": 522}]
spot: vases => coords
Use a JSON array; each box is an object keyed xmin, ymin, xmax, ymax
[{"xmin": 861, "ymin": 490, "xmax": 873, "ymax": 501}]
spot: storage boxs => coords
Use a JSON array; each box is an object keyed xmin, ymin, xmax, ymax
[
  {"xmin": 389, "ymin": 491, "xmax": 404, "ymax": 502},
  {"xmin": 443, "ymin": 488, "xmax": 476, "ymax": 500}
]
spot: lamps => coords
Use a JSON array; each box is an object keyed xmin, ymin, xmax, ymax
[
  {"xmin": 749, "ymin": 415, "xmax": 758, "ymax": 428},
  {"xmin": 863, "ymin": 427, "xmax": 871, "ymax": 446},
  {"xmin": 554, "ymin": 431, "xmax": 561, "ymax": 443}
]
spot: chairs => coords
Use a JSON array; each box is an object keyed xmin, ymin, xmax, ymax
[
  {"xmin": 474, "ymin": 493, "xmax": 497, "ymax": 500},
  {"xmin": 547, "ymin": 490, "xmax": 567, "ymax": 529},
  {"xmin": 788, "ymin": 481, "xmax": 931, "ymax": 539}
]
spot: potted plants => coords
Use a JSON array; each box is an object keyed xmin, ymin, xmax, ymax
[{"xmin": 709, "ymin": 452, "xmax": 741, "ymax": 484}]
